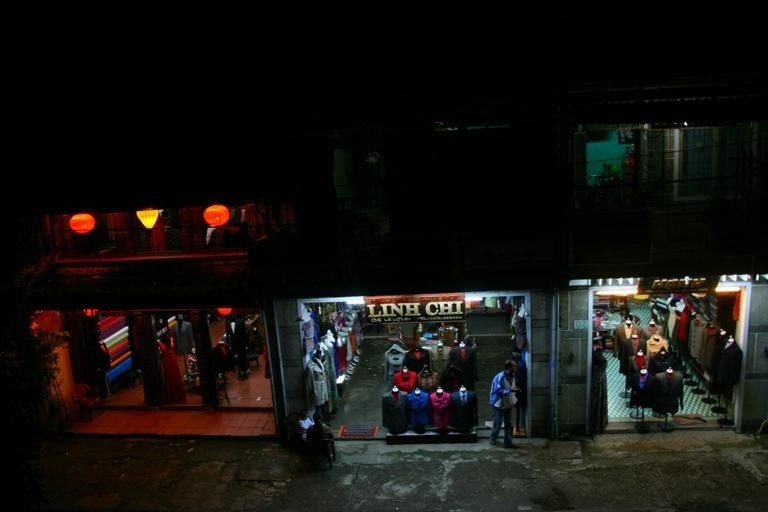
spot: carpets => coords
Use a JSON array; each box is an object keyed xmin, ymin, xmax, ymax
[{"xmin": 337, "ymin": 424, "xmax": 378, "ymax": 440}]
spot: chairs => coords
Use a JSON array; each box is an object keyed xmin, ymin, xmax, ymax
[{"xmin": 305, "ymin": 439, "xmax": 336, "ymax": 471}]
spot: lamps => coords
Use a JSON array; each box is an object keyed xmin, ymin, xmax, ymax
[{"xmin": 137, "ymin": 209, "xmax": 159, "ymax": 228}]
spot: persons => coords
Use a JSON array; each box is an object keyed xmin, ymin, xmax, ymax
[
  {"xmin": 612, "ymin": 302, "xmax": 744, "ymax": 416},
  {"xmin": 380, "ymin": 331, "xmax": 479, "ymax": 436},
  {"xmin": 490, "ymin": 359, "xmax": 521, "ymax": 449},
  {"xmin": 305, "ymin": 310, "xmax": 363, "ymax": 419},
  {"xmin": 295, "ymin": 407, "xmax": 315, "ymax": 440},
  {"xmin": 306, "ymin": 412, "xmax": 333, "ymax": 453}
]
